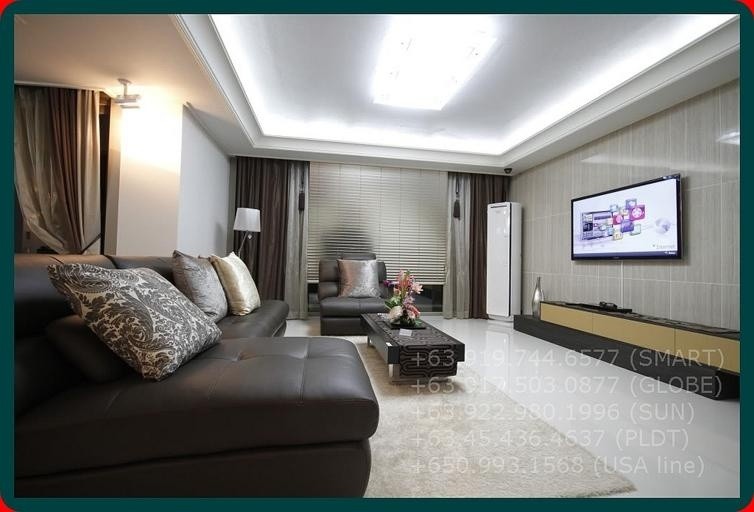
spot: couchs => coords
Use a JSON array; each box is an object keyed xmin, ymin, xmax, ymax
[{"xmin": 12, "ymin": 255, "xmax": 380, "ymax": 497}]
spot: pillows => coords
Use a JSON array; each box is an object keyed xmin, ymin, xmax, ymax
[{"xmin": 45, "ymin": 251, "xmax": 263, "ymax": 385}]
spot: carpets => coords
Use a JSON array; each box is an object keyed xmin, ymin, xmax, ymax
[{"xmin": 284, "ymin": 319, "xmax": 636, "ymax": 498}]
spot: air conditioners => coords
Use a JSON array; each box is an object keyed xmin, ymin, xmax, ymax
[{"xmin": 485, "ymin": 201, "xmax": 523, "ymax": 322}]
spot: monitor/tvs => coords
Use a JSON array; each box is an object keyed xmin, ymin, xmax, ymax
[{"xmin": 570, "ymin": 173, "xmax": 683, "ymax": 261}]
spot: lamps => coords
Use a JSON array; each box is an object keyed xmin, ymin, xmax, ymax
[{"xmin": 232, "ymin": 206, "xmax": 262, "ymax": 256}]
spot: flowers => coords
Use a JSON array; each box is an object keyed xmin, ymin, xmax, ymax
[{"xmin": 378, "ymin": 268, "xmax": 425, "ymax": 327}]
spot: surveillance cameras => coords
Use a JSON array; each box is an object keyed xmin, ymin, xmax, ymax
[{"xmin": 503, "ymin": 167, "xmax": 513, "ymax": 174}]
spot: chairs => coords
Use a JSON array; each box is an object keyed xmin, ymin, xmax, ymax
[
  {"xmin": 336, "ymin": 258, "xmax": 380, "ymax": 299},
  {"xmin": 316, "ymin": 252, "xmax": 394, "ymax": 336}
]
